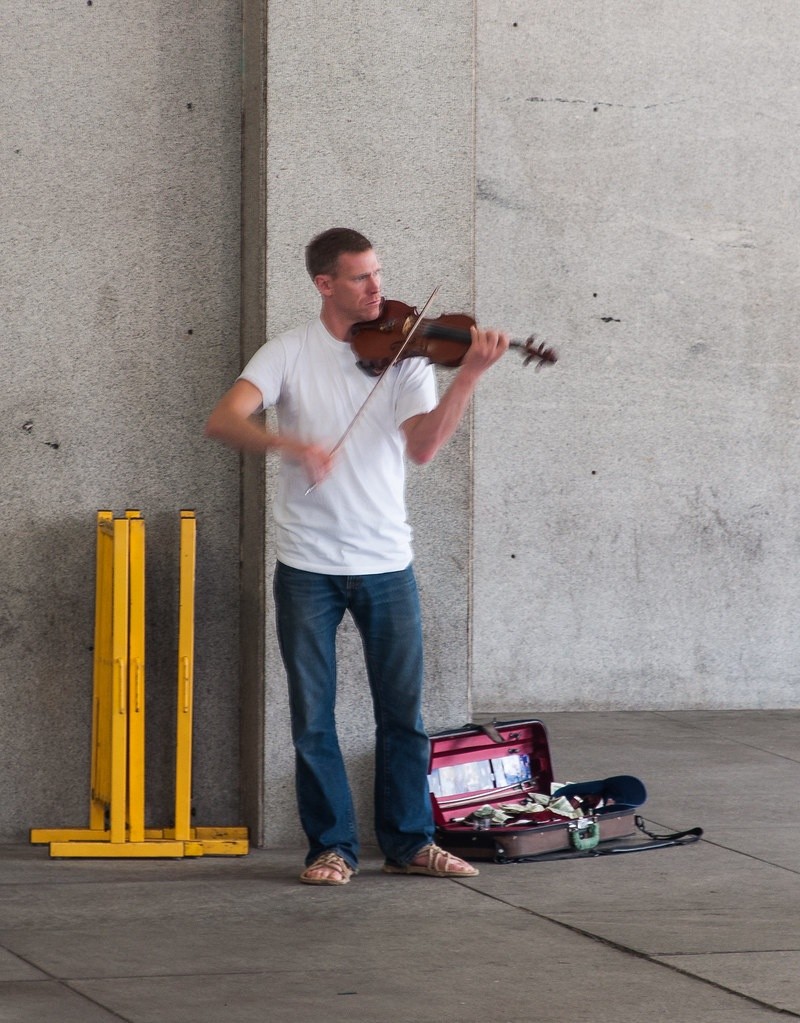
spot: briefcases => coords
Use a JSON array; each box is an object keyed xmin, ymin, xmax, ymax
[{"xmin": 422, "ymin": 717, "xmax": 705, "ymax": 862}]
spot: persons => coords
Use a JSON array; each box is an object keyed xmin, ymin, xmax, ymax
[{"xmin": 202, "ymin": 226, "xmax": 510, "ymax": 884}]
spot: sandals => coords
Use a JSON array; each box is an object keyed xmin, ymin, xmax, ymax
[
  {"xmin": 299, "ymin": 850, "xmax": 358, "ymax": 885},
  {"xmin": 383, "ymin": 842, "xmax": 479, "ymax": 877}
]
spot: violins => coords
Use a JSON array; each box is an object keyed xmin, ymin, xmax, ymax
[{"xmin": 349, "ymin": 299, "xmax": 559, "ymax": 378}]
